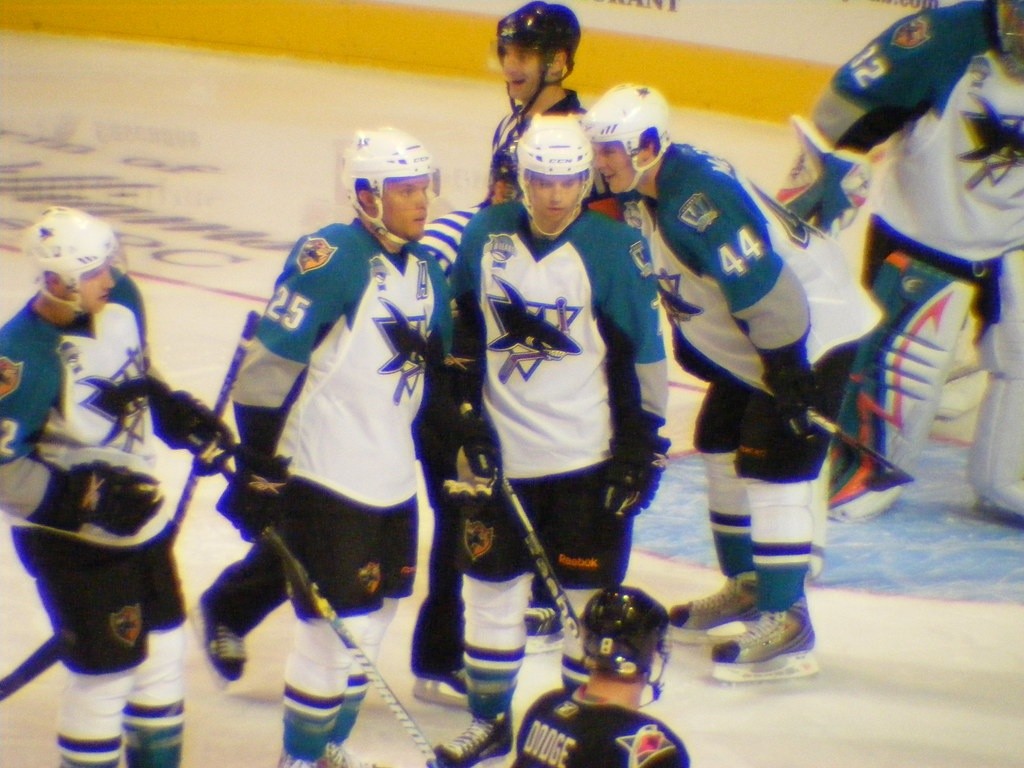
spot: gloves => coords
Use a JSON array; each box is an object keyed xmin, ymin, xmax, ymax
[
  {"xmin": 153, "ymin": 392, "xmax": 234, "ymax": 475},
  {"xmin": 431, "ymin": 423, "xmax": 501, "ymax": 511},
  {"xmin": 64, "ymin": 461, "xmax": 162, "ymax": 536},
  {"xmin": 214, "ymin": 454, "xmax": 291, "ymax": 545},
  {"xmin": 763, "ymin": 328, "xmax": 821, "ymax": 441},
  {"xmin": 601, "ymin": 434, "xmax": 672, "ymax": 514}
]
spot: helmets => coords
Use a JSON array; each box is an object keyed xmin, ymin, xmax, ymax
[
  {"xmin": 343, "ymin": 129, "xmax": 434, "ymax": 208},
  {"xmin": 25, "ymin": 204, "xmax": 118, "ymax": 287},
  {"xmin": 497, "ymin": 0, "xmax": 581, "ymax": 59},
  {"xmin": 582, "ymin": 81, "xmax": 674, "ymax": 165},
  {"xmin": 579, "ymin": 583, "xmax": 669, "ymax": 683},
  {"xmin": 516, "ymin": 113, "xmax": 593, "ymax": 199}
]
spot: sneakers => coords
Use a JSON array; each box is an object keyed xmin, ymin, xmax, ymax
[
  {"xmin": 665, "ymin": 579, "xmax": 764, "ymax": 645},
  {"xmin": 278, "ymin": 740, "xmax": 401, "ymax": 768},
  {"xmin": 415, "ymin": 664, "xmax": 471, "ymax": 709},
  {"xmin": 192, "ymin": 595, "xmax": 246, "ymax": 689},
  {"xmin": 426, "ymin": 710, "xmax": 516, "ymax": 768},
  {"xmin": 709, "ymin": 597, "xmax": 818, "ymax": 682}
]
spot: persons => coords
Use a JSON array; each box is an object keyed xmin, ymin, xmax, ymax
[
  {"xmin": 773, "ymin": 0, "xmax": 1024, "ymax": 526},
  {"xmin": 190, "ymin": 0, "xmax": 883, "ymax": 768},
  {"xmin": 0, "ymin": 206, "xmax": 235, "ymax": 768}
]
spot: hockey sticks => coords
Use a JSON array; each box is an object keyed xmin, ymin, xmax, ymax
[
  {"xmin": 1, "ymin": 309, "xmax": 260, "ymax": 701},
  {"xmin": 221, "ymin": 461, "xmax": 443, "ymax": 768},
  {"xmin": 805, "ymin": 408, "xmax": 917, "ymax": 494},
  {"xmin": 501, "ymin": 473, "xmax": 579, "ymax": 639}
]
